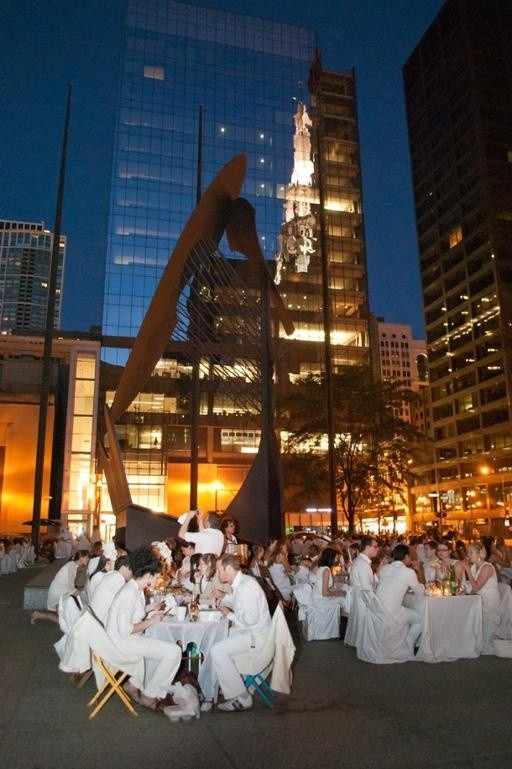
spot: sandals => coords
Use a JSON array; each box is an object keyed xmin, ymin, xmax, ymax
[
  {"xmin": 122, "ymin": 680, "xmax": 141, "ymax": 704},
  {"xmin": 139, "ymin": 697, "xmax": 167, "ymax": 712}
]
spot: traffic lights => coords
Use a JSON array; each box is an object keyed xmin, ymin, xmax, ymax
[{"xmin": 463, "ymin": 494, "xmax": 469, "ymax": 510}]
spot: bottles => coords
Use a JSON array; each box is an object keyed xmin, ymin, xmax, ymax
[
  {"xmin": 190, "ymin": 592, "xmax": 198, "ymax": 622},
  {"xmin": 442, "ymin": 567, "xmax": 449, "ymax": 595},
  {"xmin": 450, "ymin": 565, "xmax": 457, "ymax": 595}
]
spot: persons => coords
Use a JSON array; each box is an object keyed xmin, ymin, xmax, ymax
[
  {"xmin": 31, "ymin": 539, "xmax": 272, "ymax": 711},
  {"xmin": 250, "ymin": 528, "xmax": 511, "ymax": 653},
  {"xmin": 1, "ymin": 525, "xmax": 100, "ymax": 573},
  {"xmin": 178, "ymin": 511, "xmax": 237, "ymax": 557}
]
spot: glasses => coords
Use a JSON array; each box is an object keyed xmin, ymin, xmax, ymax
[{"xmin": 436, "ymin": 548, "xmax": 449, "ymax": 552}]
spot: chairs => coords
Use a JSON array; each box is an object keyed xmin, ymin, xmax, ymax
[
  {"xmin": 0, "ymin": 540, "xmax": 36, "ymax": 574},
  {"xmin": 73, "ymin": 608, "xmax": 143, "ymax": 719},
  {"xmin": 62, "ymin": 551, "xmax": 280, "ymax": 635},
  {"xmin": 308, "ymin": 573, "xmax": 511, "ymax": 663},
  {"xmin": 53, "ymin": 530, "xmax": 92, "ymax": 559},
  {"xmin": 230, "ymin": 601, "xmax": 299, "ymax": 713}
]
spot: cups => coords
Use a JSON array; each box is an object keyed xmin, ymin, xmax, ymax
[{"xmin": 175, "ymin": 606, "xmax": 186, "ymax": 622}]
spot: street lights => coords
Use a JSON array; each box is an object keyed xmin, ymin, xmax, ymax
[{"xmin": 482, "ymin": 466, "xmax": 492, "ymax": 536}]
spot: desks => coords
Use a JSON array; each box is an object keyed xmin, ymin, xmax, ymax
[{"xmin": 137, "ymin": 573, "xmax": 230, "ymax": 698}]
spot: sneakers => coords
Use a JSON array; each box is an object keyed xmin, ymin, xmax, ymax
[{"xmin": 217, "ymin": 694, "xmax": 254, "ymax": 713}]
